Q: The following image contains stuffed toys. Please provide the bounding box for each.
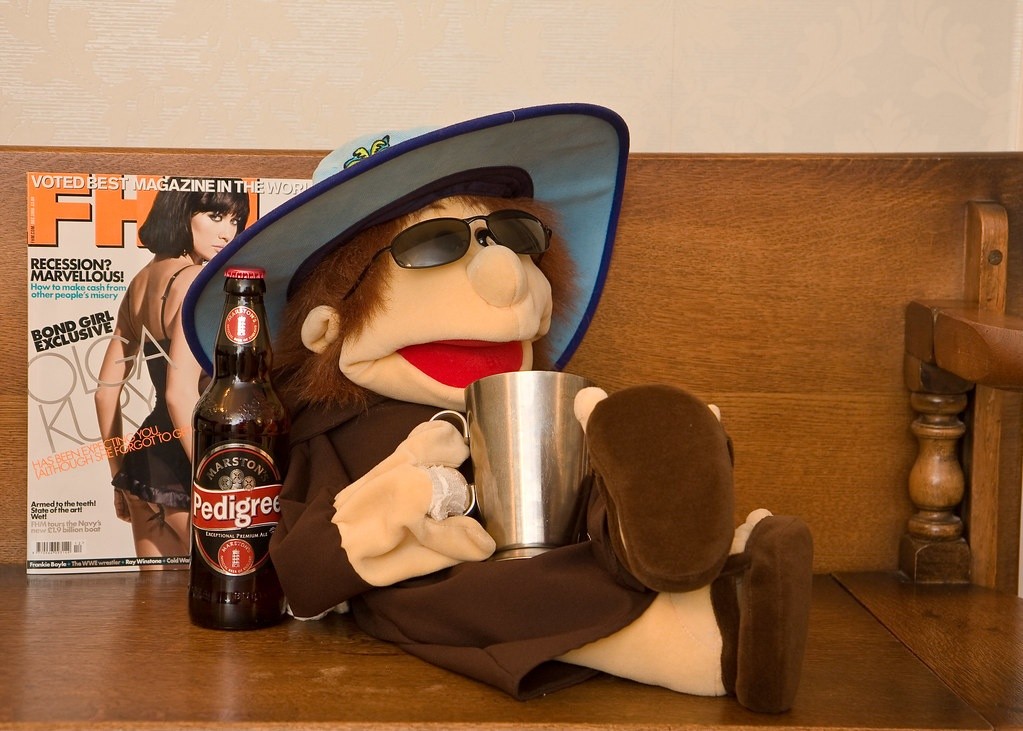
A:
[181,102,814,712]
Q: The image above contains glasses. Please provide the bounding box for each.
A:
[343,208,553,304]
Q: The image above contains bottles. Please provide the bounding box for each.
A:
[189,267,287,627]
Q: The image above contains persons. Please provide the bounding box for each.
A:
[95,177,251,571]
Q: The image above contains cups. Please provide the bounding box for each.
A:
[429,371,586,562]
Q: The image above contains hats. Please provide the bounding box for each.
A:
[180,104,630,379]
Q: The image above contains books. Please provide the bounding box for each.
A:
[27,171,311,574]
